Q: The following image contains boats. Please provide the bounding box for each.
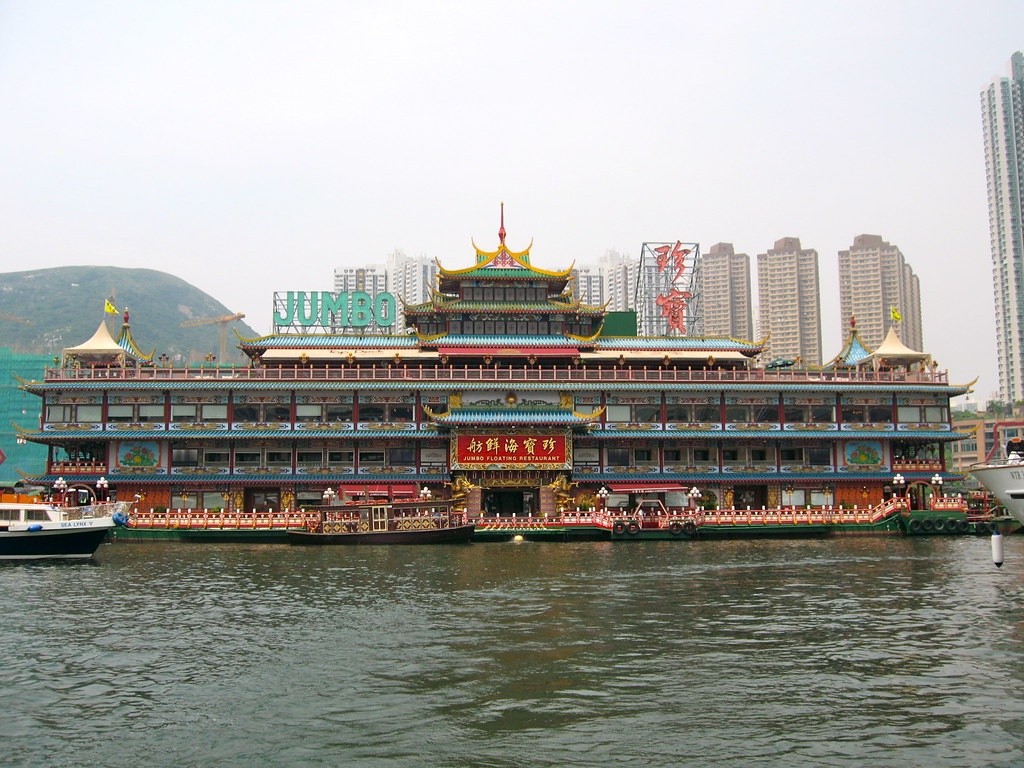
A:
[0,481,137,561]
[966,422,1024,526]
[286,463,475,545]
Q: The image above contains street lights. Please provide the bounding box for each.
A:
[688,487,703,513]
[322,486,335,505]
[53,477,68,506]
[96,477,109,500]
[931,474,943,499]
[893,473,905,499]
[596,487,610,512]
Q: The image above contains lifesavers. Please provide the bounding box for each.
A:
[613,521,626,534]
[113,512,126,525]
[958,520,969,531]
[627,522,639,535]
[988,522,999,533]
[683,523,696,536]
[976,522,987,534]
[946,518,958,531]
[922,519,933,531]
[909,519,921,530]
[934,519,945,530]
[670,523,682,534]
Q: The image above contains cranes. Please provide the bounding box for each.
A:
[178,314,243,363]
